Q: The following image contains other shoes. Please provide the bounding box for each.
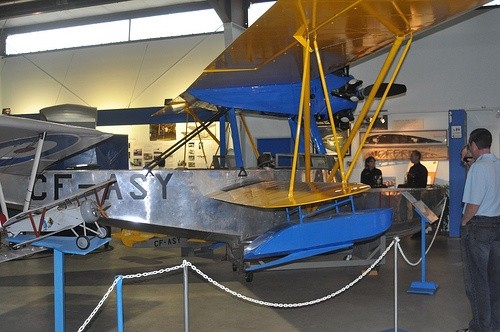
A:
[411,234,420,240]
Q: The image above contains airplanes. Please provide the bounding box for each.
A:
[0,0,492,284]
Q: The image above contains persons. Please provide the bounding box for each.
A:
[457,128,500,332]
[360,156,387,188]
[398,150,427,239]
